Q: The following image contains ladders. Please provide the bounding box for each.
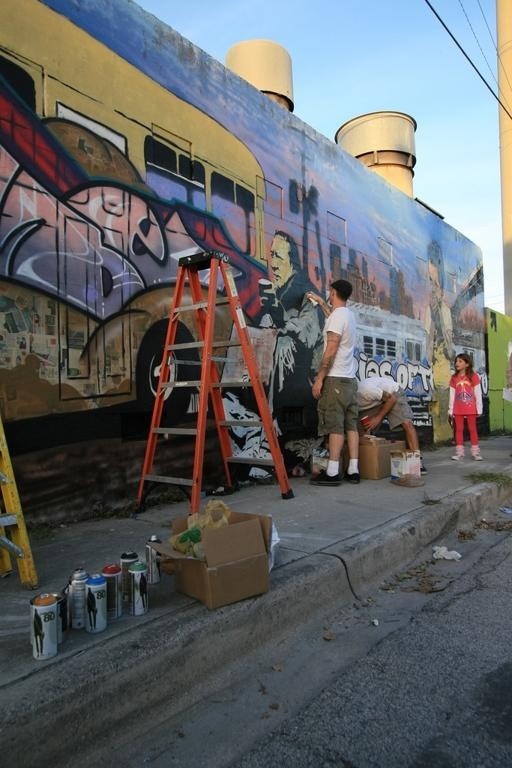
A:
[0,420,36,589]
[133,250,296,514]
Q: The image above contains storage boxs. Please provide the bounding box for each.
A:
[146,511,273,609]
[325,439,422,480]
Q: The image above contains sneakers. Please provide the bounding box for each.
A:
[344,469,360,484]
[310,469,342,486]
[421,465,427,474]
[474,454,483,460]
[452,453,464,460]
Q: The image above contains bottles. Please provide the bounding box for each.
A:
[28,529,163,660]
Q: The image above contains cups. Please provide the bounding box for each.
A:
[361,415,372,431]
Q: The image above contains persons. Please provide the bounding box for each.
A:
[356,377,429,475]
[257,229,326,442]
[446,352,485,461]
[423,239,458,367]
[305,279,361,486]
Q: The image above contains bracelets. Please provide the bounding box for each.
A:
[319,302,326,307]
